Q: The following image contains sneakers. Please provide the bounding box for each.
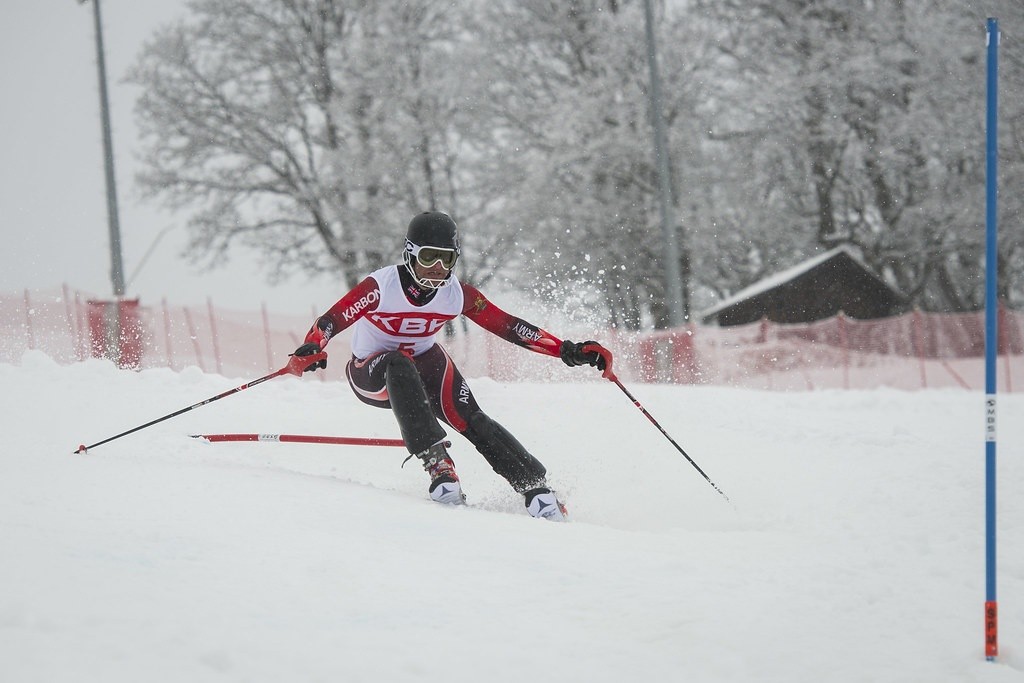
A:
[520,477,568,522]
[415,440,468,506]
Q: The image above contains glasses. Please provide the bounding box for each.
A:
[402,235,461,270]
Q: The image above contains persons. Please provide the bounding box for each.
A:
[287,209,612,524]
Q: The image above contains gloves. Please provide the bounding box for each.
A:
[560,340,607,371]
[288,344,327,372]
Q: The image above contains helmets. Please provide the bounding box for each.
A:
[405,211,459,267]
